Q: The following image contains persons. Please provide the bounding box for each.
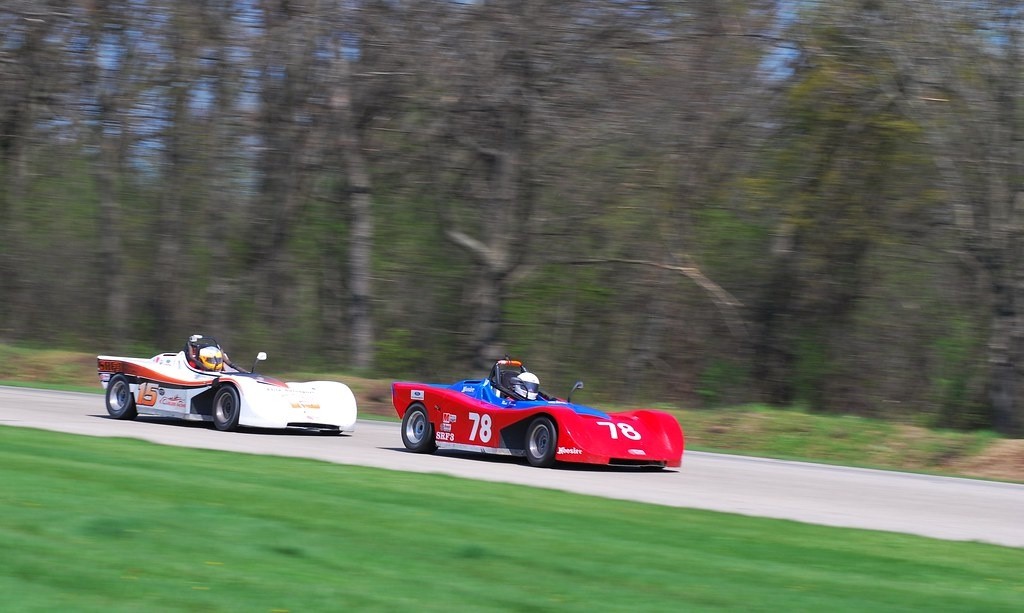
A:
[196,347,223,372]
[505,372,540,401]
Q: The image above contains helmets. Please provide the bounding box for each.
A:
[515,372,540,401]
[200,346,223,371]
